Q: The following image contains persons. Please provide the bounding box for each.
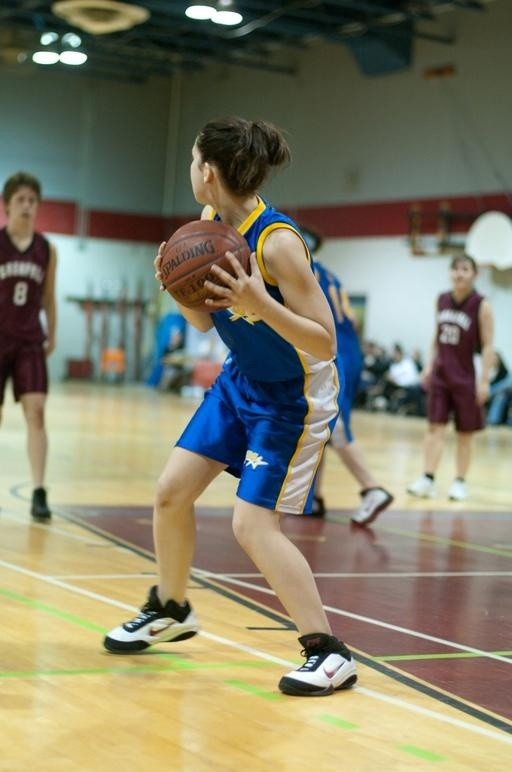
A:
[159,329,226,395]
[284,222,393,529]
[277,508,391,612]
[354,340,511,430]
[0,169,59,520]
[102,116,360,696]
[405,253,495,503]
[0,515,57,556]
[408,492,466,656]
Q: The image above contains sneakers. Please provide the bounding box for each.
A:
[104,583,201,654]
[407,476,437,499]
[294,494,327,518]
[32,488,53,521]
[447,482,467,502]
[276,631,358,697]
[351,485,394,529]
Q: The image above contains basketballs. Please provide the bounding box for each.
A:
[158,221,251,310]
